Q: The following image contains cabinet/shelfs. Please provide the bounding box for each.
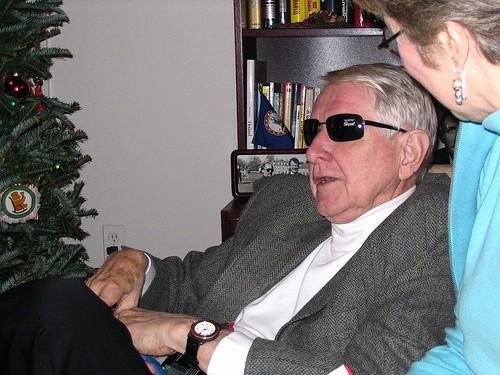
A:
[221,0,459,245]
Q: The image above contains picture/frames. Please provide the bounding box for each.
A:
[231,148,308,200]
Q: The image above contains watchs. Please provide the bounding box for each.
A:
[185,318,220,368]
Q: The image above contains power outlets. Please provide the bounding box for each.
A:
[102,224,126,262]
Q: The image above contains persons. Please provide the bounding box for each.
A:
[285,158,303,176]
[253,163,274,190]
[243,165,247,179]
[237,163,242,183]
[0,62,456,375]
[345,0,500,375]
[433,110,460,166]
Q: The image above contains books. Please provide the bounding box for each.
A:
[248,0,380,30]
[247,59,320,150]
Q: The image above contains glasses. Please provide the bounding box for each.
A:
[378,27,415,58]
[303,114,409,146]
